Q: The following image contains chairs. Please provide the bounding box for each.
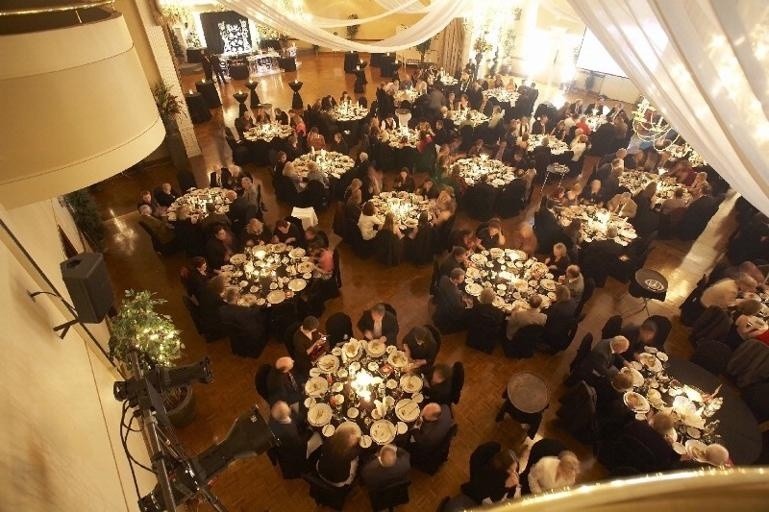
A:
[242,301,472,512]
[425,214,601,361]
[135,66,769,512]
[560,197,766,483]
[469,440,516,489]
[518,434,567,477]
[182,66,737,285]
[141,158,271,263]
[176,208,352,362]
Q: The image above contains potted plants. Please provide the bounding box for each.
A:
[107,287,195,429]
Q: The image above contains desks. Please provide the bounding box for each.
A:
[227,52,296,71]
[344,51,402,82]
[500,367,554,423]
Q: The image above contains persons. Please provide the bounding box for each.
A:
[582,320,731,470]
[138,51,629,339]
[438,448,580,510]
[628,114,713,232]
[701,261,768,350]
[267,315,453,500]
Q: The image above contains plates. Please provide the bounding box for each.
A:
[405,212,433,229]
[463,248,559,315]
[618,350,712,464]
[219,245,315,307]
[743,289,769,331]
[617,221,638,240]
[305,339,425,450]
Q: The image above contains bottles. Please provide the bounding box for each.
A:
[703,397,724,418]
[703,420,720,436]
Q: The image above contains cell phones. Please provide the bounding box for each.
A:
[319,336,327,341]
